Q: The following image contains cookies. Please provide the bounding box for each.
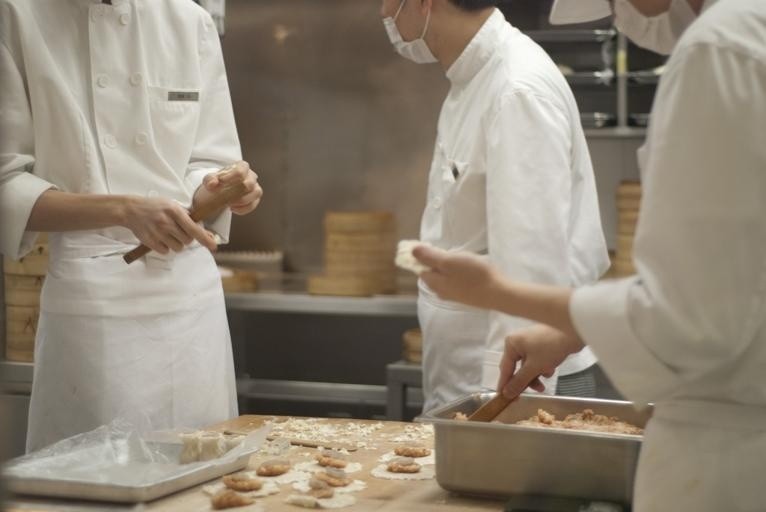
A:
[211,445,430,510]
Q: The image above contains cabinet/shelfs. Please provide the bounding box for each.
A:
[521,26,665,137]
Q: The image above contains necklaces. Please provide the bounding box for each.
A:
[410,2,766,510]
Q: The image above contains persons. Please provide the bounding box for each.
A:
[0,0,266,463]
[377,1,611,421]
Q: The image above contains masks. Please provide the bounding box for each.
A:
[382,0,440,64]
[612,0,677,56]
